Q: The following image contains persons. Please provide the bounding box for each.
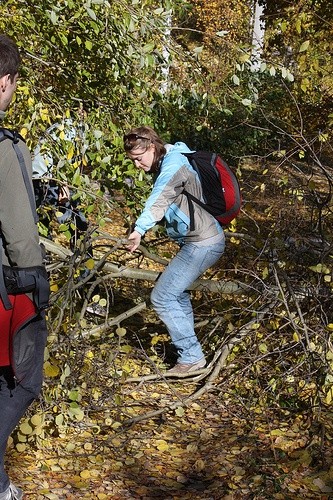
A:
[122,127,225,373]
[0,33,104,500]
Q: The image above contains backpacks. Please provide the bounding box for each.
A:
[158,149,242,226]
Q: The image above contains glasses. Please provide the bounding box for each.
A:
[123,134,153,143]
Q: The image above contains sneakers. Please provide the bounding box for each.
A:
[167,359,206,373]
[9,484,23,500]
[86,303,107,317]
